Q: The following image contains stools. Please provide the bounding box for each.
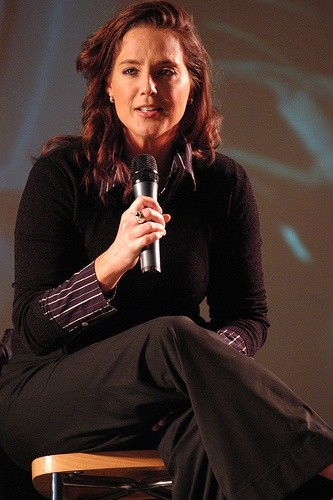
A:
[31,449,165,500]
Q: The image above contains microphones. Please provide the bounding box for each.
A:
[131,154,162,273]
